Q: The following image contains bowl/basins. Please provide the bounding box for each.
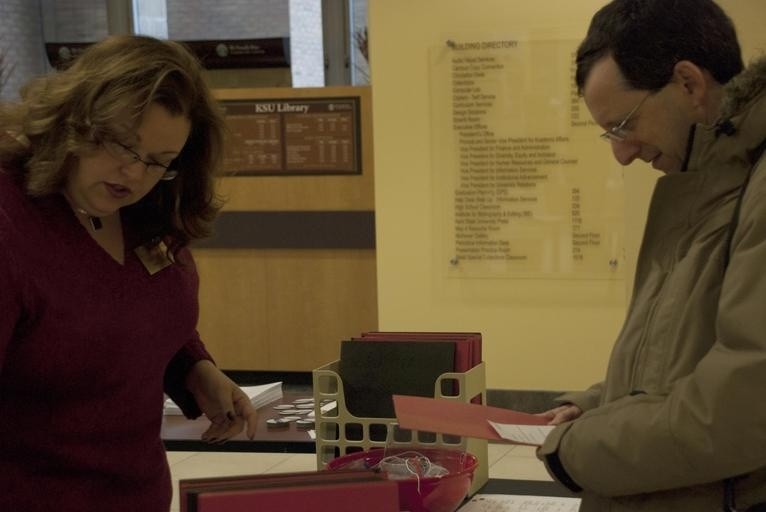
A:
[326,446,478,511]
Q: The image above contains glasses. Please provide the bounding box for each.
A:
[100,137,178,181]
[600,90,650,142]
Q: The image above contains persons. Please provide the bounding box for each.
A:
[530,0,766,512]
[0,34,260,511]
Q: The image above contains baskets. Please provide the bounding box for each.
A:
[312,359,489,499]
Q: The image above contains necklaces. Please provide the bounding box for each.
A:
[70,202,103,232]
